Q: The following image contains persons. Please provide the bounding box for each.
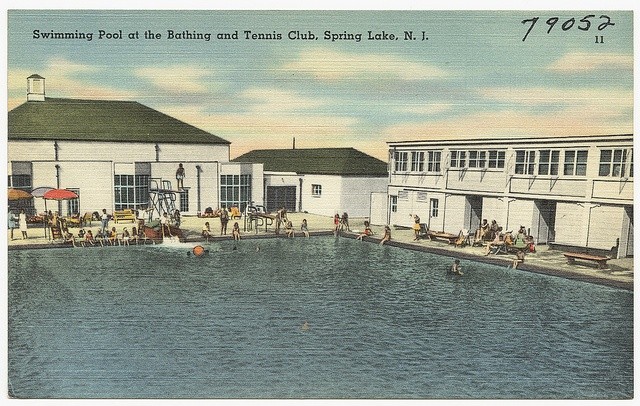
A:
[176,164,185,192]
[276,206,287,234]
[47,210,52,231]
[99,209,109,229]
[257,247,260,252]
[220,205,229,235]
[490,220,498,231]
[202,222,211,243]
[356,221,373,241]
[204,250,209,255]
[186,252,191,257]
[131,227,137,245]
[233,223,240,241]
[19,209,27,239]
[8,209,15,241]
[515,226,535,252]
[334,213,341,231]
[483,236,504,256]
[78,229,86,247]
[61,229,76,247]
[450,260,459,272]
[503,230,514,244]
[105,230,112,246]
[341,212,352,233]
[513,249,524,269]
[481,219,489,232]
[111,227,121,246]
[174,210,180,228]
[285,221,294,238]
[494,227,503,241]
[301,219,309,237]
[95,228,105,247]
[130,205,155,232]
[158,212,173,239]
[379,225,391,245]
[86,230,95,246]
[122,227,131,247]
[413,215,420,236]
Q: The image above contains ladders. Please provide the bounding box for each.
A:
[51,225,63,239]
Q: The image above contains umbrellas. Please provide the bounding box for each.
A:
[31,186,56,213]
[43,188,79,216]
[8,188,34,201]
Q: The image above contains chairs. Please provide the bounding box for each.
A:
[80,211,93,226]
[453,228,471,247]
[231,205,242,220]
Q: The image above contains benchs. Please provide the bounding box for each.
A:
[113,208,136,223]
[563,251,611,269]
[426,230,460,245]
